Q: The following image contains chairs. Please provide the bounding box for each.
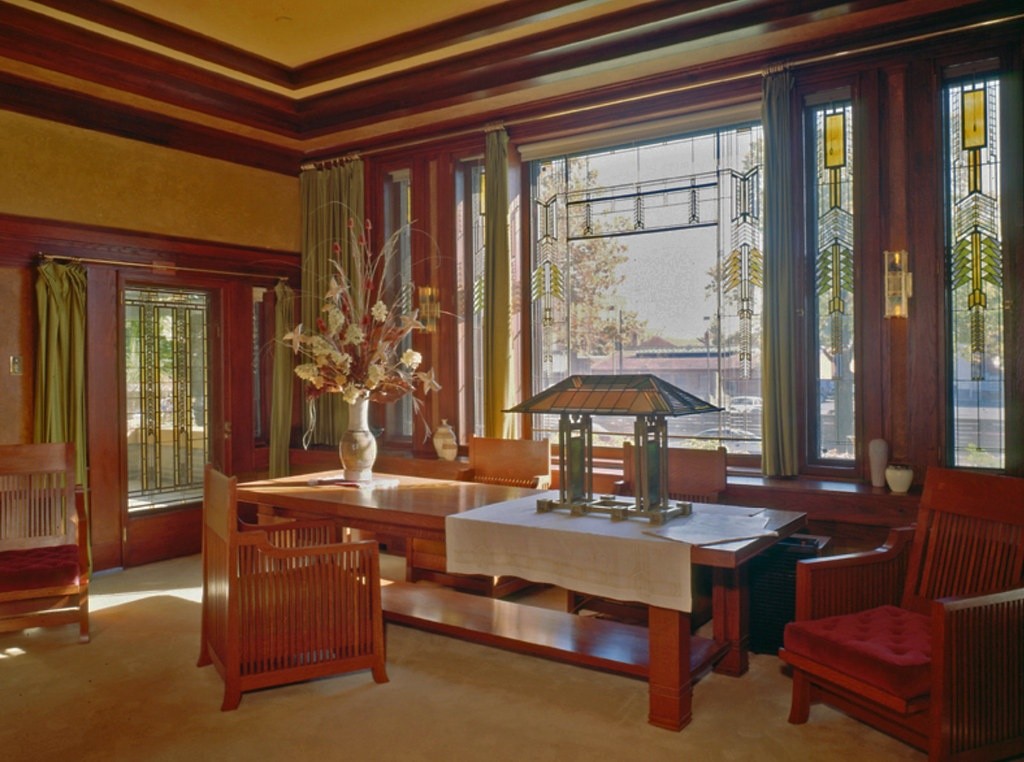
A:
[194,461,390,713]
[778,467,1024,762]
[565,442,728,637]
[0,442,92,646]
[405,432,553,599]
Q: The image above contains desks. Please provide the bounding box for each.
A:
[235,469,811,733]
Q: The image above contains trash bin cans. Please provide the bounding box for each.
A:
[749,536,820,655]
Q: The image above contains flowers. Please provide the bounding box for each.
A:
[279,201,463,451]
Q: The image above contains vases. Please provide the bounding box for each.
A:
[339,389,379,481]
[885,464,914,493]
[870,438,888,487]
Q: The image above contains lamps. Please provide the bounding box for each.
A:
[417,286,438,334]
[883,250,913,318]
